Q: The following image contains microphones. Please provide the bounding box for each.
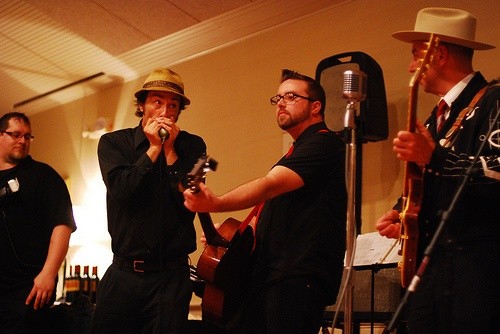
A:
[342,69,368,100]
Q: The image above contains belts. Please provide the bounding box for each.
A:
[113,253,151,273]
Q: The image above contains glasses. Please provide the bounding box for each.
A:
[4,131,34,142]
[270,91,314,105]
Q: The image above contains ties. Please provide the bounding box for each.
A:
[435,100,448,134]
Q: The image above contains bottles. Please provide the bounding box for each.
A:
[373,323,386,334]
[64,265,100,304]
[359,323,371,334]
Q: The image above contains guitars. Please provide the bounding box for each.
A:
[397,33,439,290]
[184,152,256,330]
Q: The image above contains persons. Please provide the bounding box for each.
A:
[92,68,206,334]
[0,112,77,334]
[183,70,348,334]
[376,9,500,334]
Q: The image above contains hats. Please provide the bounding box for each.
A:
[135,68,190,108]
[391,7,496,55]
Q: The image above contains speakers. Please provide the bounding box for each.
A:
[315,51,388,145]
